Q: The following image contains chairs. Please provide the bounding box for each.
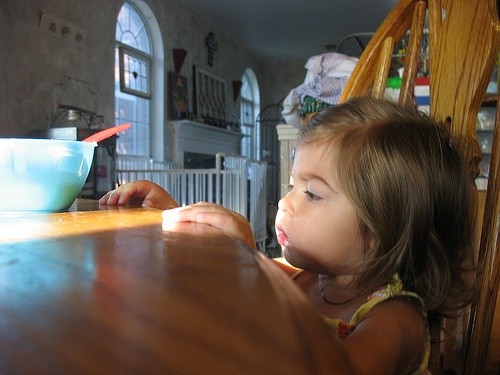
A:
[338,0,500,375]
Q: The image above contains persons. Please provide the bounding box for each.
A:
[98,97,479,375]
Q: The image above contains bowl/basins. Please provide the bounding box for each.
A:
[0,138,94,211]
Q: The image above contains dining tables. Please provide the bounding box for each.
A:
[0,197,352,375]
